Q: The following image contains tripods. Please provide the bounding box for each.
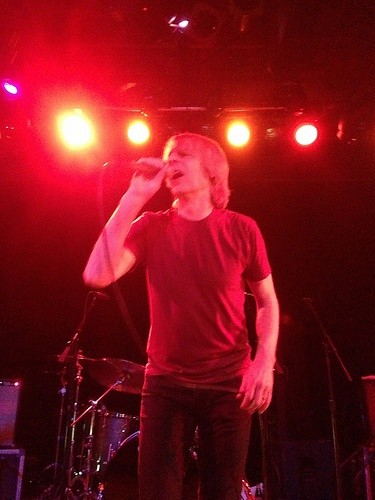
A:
[38,301,129,500]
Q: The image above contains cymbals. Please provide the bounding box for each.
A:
[85,359,146,394]
[56,354,94,364]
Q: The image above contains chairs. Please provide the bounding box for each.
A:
[0,382,25,500]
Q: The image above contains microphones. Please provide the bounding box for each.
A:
[303,297,327,337]
[106,161,174,177]
[89,291,111,301]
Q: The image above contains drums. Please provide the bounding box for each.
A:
[65,400,106,459]
[110,431,201,500]
[93,410,140,464]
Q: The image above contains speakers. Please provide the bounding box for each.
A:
[0,449,25,500]
[0,381,21,449]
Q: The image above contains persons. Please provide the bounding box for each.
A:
[83,133,279,500]
